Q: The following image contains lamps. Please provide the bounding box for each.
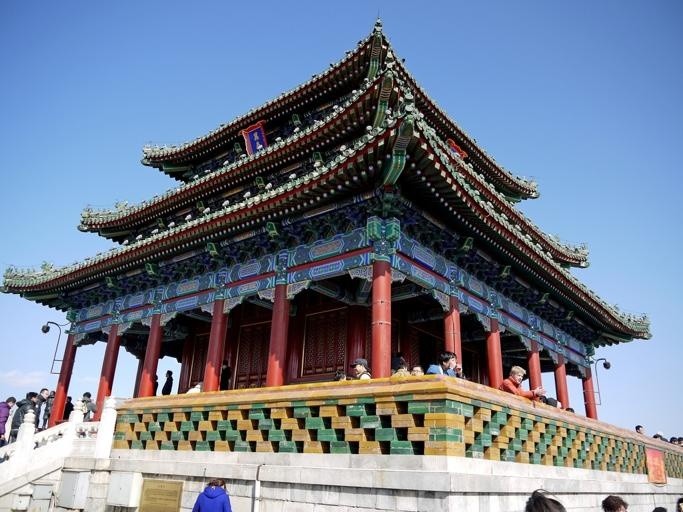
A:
[590,356,610,406]
[41,321,72,374]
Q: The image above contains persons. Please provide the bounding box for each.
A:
[191,476,234,512]
[219,360,232,390]
[523,487,567,512]
[652,434,661,440]
[154,375,160,397]
[675,497,683,512]
[337,357,372,381]
[0,385,102,463]
[677,437,682,447]
[599,494,630,512]
[161,370,174,396]
[390,351,578,413]
[634,425,644,435]
[669,437,677,446]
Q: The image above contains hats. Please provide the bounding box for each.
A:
[350,358,368,368]
